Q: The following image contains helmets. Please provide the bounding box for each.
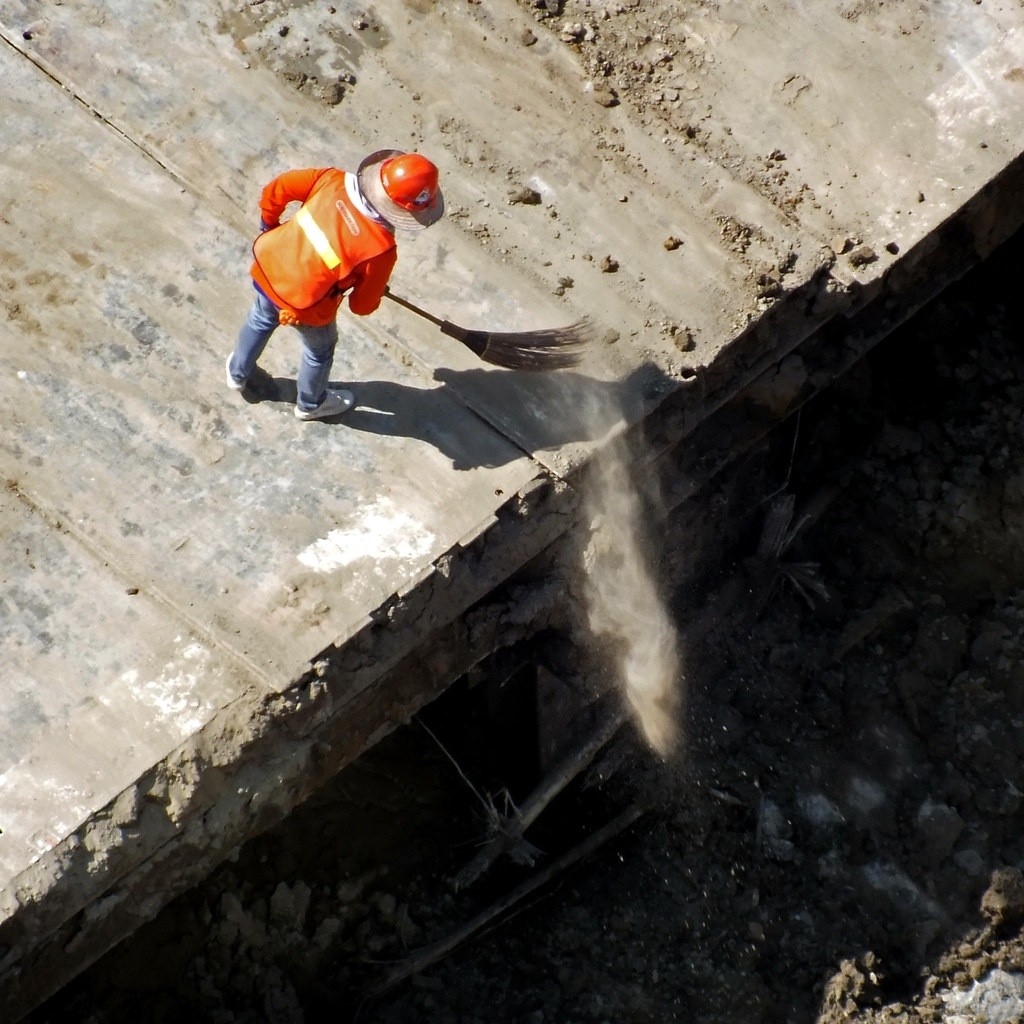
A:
[381,153,438,211]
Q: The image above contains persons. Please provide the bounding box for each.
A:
[223,147,444,421]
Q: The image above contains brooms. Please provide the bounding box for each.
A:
[382,289,594,374]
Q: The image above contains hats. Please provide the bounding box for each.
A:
[356,147,444,231]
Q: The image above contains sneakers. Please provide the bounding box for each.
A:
[293,389,355,421]
[226,351,246,392]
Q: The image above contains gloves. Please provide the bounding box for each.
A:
[261,218,281,232]
